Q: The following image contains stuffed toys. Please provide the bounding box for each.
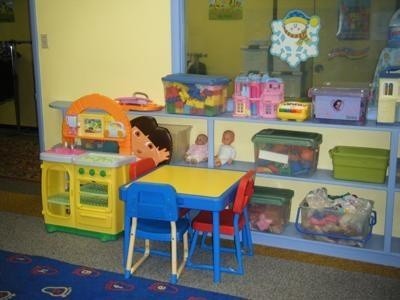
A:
[253,143,312,178]
[249,203,291,234]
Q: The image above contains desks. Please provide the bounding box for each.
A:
[119,164,248,283]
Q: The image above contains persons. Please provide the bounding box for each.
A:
[214,130,236,167]
[184,133,209,164]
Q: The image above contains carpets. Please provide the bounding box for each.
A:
[0,248,249,300]
[0,131,41,183]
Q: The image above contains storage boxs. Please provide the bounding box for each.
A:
[329,144,390,184]
[155,123,192,163]
[162,41,369,124]
[251,129,323,179]
[294,194,376,248]
[246,185,294,234]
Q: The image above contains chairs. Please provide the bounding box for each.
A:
[122,181,190,285]
[186,171,255,275]
[1,44,22,131]
[130,158,198,224]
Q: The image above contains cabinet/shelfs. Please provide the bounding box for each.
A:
[49,101,400,279]
